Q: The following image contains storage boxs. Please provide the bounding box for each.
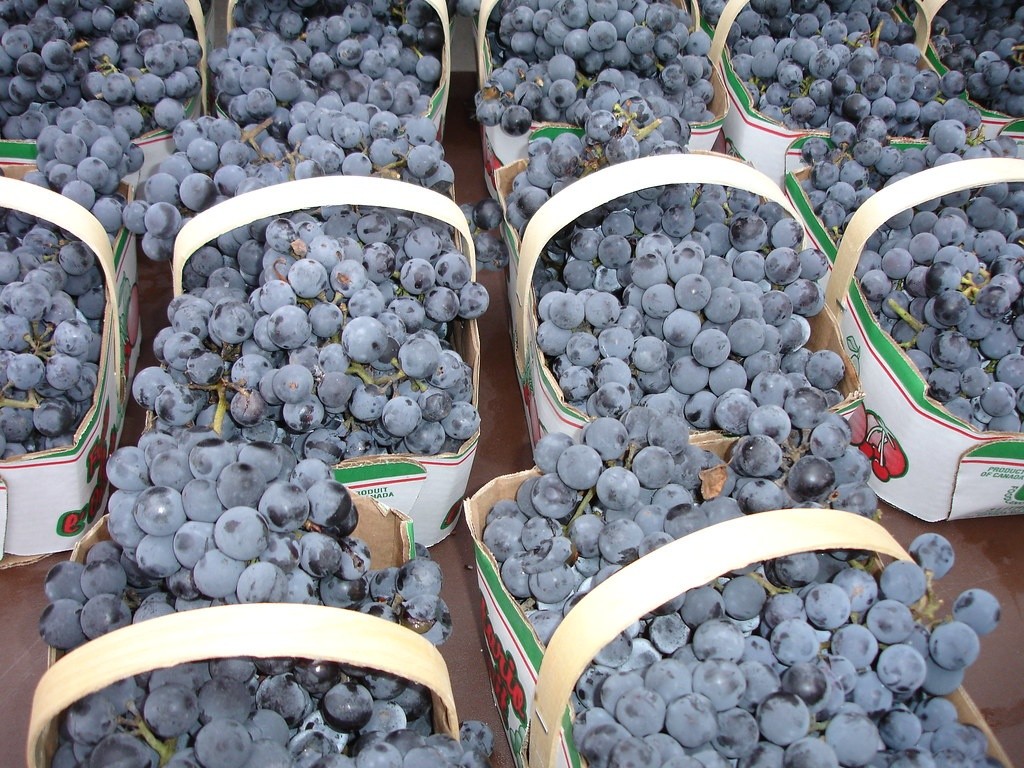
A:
[1,0,1023,767]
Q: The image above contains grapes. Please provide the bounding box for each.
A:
[0,0,1024,768]
